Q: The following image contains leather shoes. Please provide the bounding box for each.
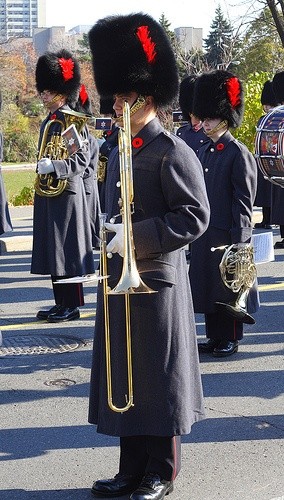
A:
[91,476,136,500]
[130,474,173,500]
[197,339,215,353]
[47,305,80,322]
[275,239,284,248]
[212,341,239,356]
[254,221,272,229]
[36,304,62,320]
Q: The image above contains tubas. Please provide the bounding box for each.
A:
[34,109,95,197]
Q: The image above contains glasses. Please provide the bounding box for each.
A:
[39,91,55,97]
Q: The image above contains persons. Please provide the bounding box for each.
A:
[0,92,13,237]
[274,71,284,249]
[178,75,209,154]
[193,69,258,357]
[253,80,277,230]
[76,83,102,248]
[29,48,91,324]
[87,11,210,500]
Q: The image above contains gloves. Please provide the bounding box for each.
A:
[37,158,54,174]
[233,243,250,251]
[104,222,125,257]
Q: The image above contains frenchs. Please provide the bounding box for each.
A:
[211,243,257,324]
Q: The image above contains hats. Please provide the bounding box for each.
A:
[194,70,244,128]
[178,75,200,113]
[88,12,179,106]
[68,83,93,116]
[99,96,116,114]
[35,50,81,103]
[272,72,284,104]
[260,79,276,106]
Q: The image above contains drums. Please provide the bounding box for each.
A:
[255,105,284,188]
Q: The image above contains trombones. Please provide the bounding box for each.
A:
[100,101,159,414]
